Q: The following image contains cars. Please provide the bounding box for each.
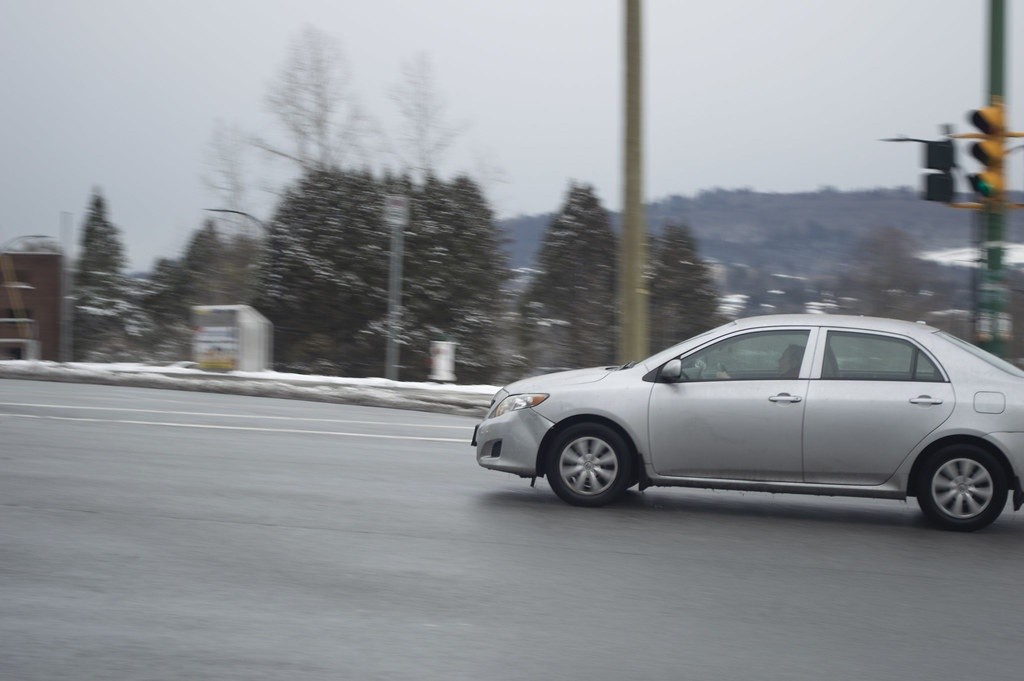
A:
[469,314,1024,532]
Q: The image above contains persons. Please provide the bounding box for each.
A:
[715,344,804,379]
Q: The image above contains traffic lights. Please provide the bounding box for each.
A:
[922,136,952,202]
[969,105,1004,198]
